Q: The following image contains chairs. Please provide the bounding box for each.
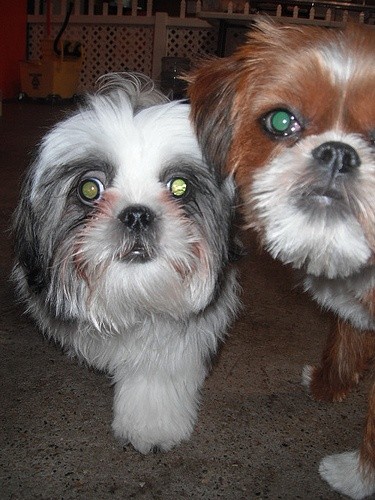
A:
[159,56,193,100]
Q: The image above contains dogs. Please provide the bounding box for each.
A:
[5,10,375,500]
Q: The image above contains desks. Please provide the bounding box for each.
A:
[196,11,345,58]
